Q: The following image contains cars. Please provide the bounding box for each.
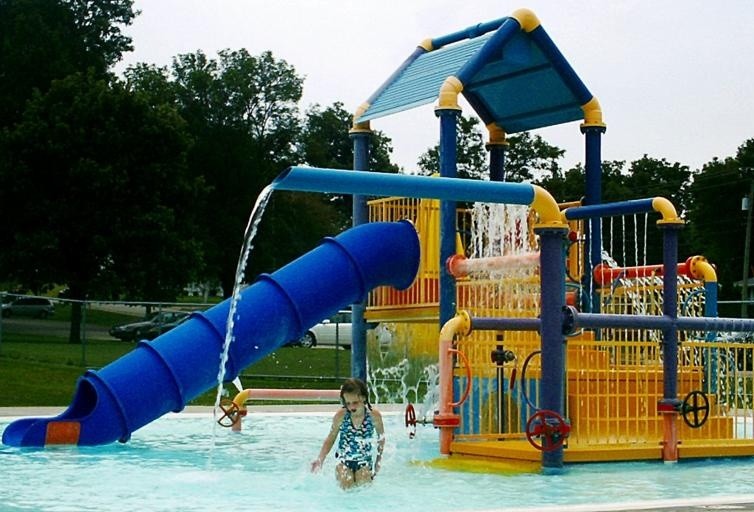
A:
[660,332,754,374]
[0,296,55,318]
[108,311,191,341]
[298,310,352,349]
[59,288,88,300]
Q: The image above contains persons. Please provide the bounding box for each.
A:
[310,377,386,491]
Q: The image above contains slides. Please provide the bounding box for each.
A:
[2,222,420,447]
